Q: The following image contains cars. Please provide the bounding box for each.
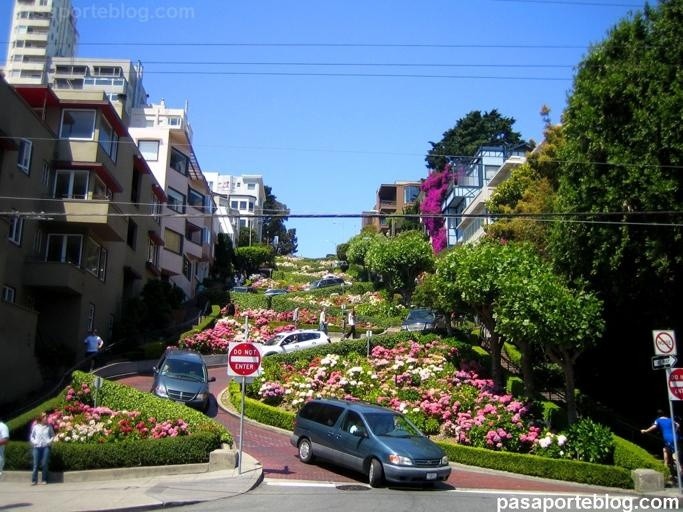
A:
[309,277,344,290]
[263,288,288,297]
[259,327,332,357]
[401,306,446,336]
[333,260,348,267]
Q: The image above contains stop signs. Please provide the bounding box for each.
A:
[226,341,262,377]
[665,366,683,401]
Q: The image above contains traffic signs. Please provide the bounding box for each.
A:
[650,354,678,372]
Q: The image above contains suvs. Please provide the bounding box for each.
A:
[149,345,217,411]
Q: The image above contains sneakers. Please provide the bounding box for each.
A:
[31,481,47,485]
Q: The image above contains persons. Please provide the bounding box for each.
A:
[318,306,330,335]
[30,411,56,485]
[351,419,367,436]
[640,410,683,475]
[84,328,104,372]
[0,417,10,473]
[293,304,301,329]
[343,308,356,339]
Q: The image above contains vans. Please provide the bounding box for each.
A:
[288,399,451,488]
